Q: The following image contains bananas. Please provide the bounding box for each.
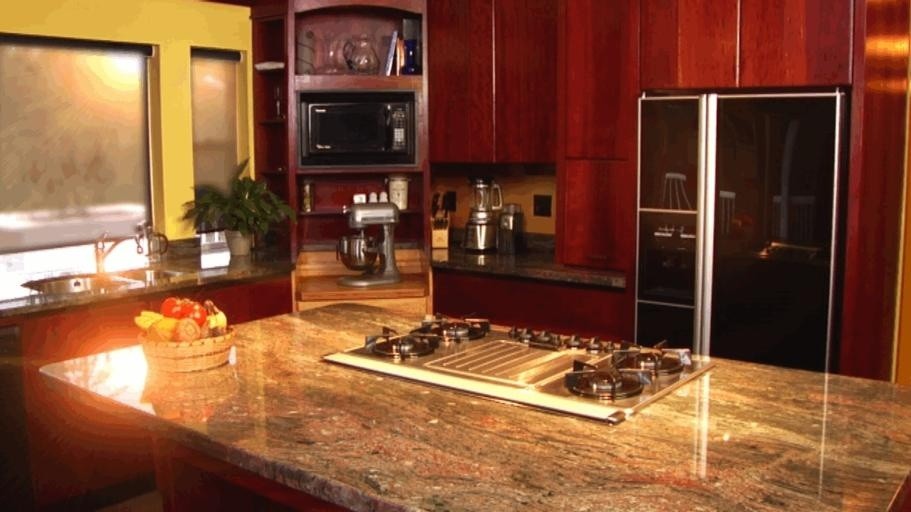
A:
[133,299,227,338]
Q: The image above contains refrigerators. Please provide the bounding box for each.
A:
[632,83,849,377]
[606,350,842,512]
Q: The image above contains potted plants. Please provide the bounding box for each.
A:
[180,156,298,256]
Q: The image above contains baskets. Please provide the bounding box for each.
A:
[139,327,236,373]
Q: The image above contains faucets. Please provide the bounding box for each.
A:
[95,231,146,271]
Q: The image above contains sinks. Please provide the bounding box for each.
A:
[22,274,127,296]
[114,266,188,284]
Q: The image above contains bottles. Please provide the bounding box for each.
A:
[499,203,521,254]
[296,30,315,74]
[403,38,419,75]
[299,178,315,212]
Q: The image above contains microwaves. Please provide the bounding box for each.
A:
[305,99,413,164]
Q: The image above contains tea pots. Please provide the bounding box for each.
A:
[342,33,379,75]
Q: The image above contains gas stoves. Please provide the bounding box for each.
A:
[321,311,718,424]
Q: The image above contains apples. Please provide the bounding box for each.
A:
[160,296,206,325]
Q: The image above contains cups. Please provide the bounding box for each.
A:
[387,175,412,210]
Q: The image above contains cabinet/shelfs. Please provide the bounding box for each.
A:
[555,1,642,273]
[639,0,851,88]
[17,273,292,510]
[253,0,433,315]
[425,1,554,166]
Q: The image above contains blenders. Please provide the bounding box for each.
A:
[460,175,504,252]
[337,201,402,287]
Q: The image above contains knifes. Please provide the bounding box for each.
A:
[431,193,452,231]
